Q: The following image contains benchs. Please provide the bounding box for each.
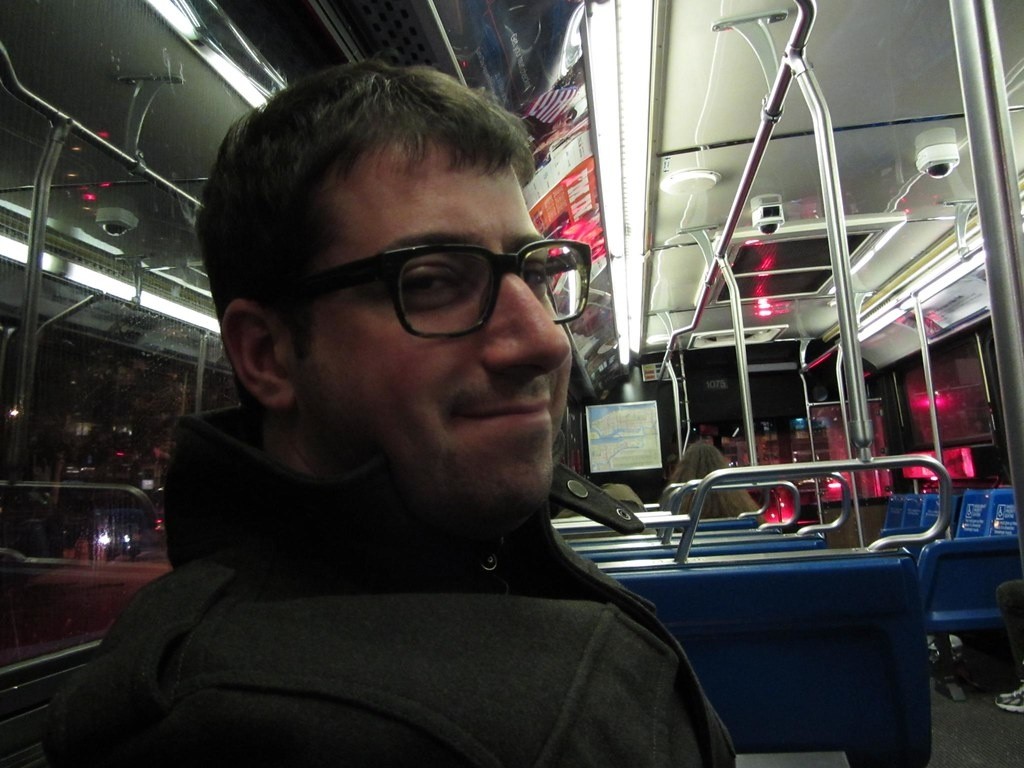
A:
[548,453,1024,767]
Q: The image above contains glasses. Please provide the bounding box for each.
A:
[270,234,593,340]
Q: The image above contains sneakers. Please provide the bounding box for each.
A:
[994,682,1024,713]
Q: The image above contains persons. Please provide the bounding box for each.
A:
[43,61,735,768]
[29,450,70,511]
[995,580,1024,714]
[663,440,764,526]
